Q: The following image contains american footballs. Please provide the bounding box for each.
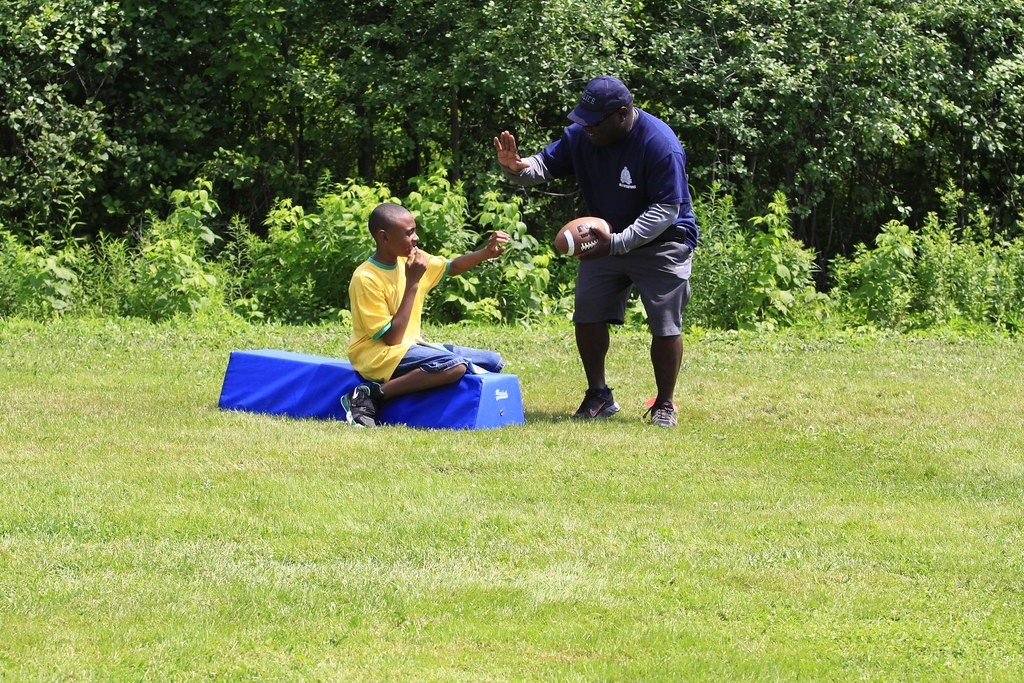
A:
[555,217,614,258]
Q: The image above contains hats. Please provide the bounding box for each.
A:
[568,75,633,126]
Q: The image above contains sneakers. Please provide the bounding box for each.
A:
[342,381,385,429]
[572,386,620,420]
[651,398,678,429]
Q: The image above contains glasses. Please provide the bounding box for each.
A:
[589,108,619,126]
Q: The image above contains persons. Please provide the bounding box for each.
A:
[339,201,509,431]
[494,75,699,429]
